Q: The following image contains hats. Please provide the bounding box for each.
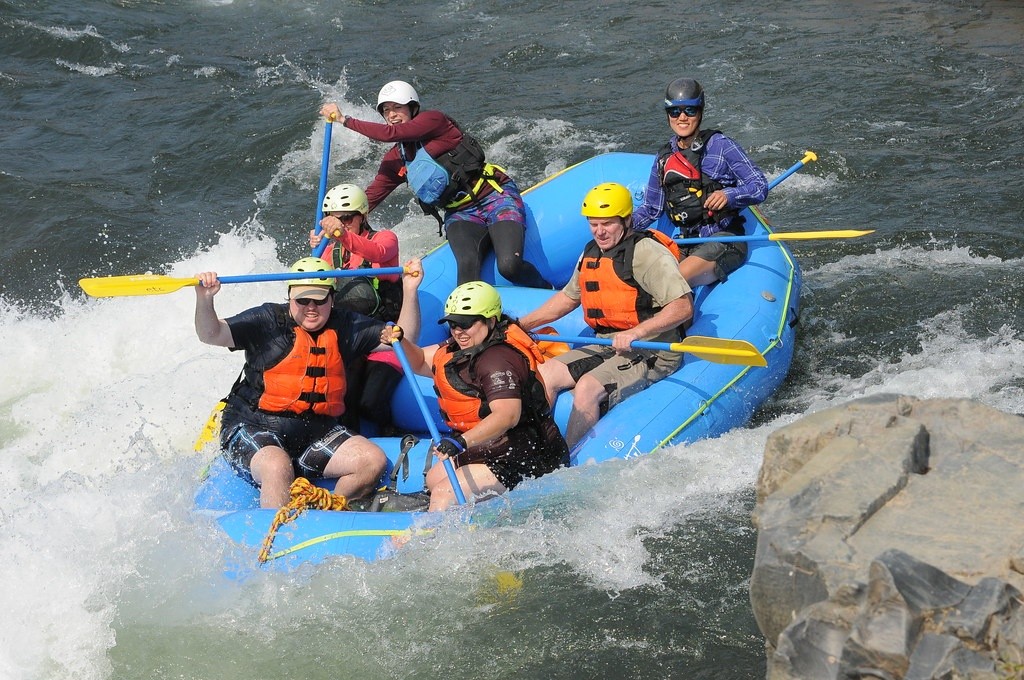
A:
[438,313,486,328]
[289,284,332,300]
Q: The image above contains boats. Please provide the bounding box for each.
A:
[189,149,805,584]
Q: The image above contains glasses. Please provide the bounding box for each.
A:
[340,212,359,225]
[449,321,481,330]
[297,297,330,306]
[665,107,701,118]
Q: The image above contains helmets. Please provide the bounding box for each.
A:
[288,257,335,291]
[444,280,501,322]
[664,77,704,109]
[582,181,633,219]
[376,80,420,117]
[322,184,369,217]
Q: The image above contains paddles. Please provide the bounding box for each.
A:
[79,266,419,298]
[528,333,767,367]
[390,324,468,508]
[768,152,818,189]
[311,113,336,259]
[313,223,340,258]
[672,229,875,244]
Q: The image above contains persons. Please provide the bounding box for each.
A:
[629,77,769,332]
[309,81,556,292]
[517,183,694,451]
[194,257,424,508]
[314,184,409,439]
[380,280,572,513]
